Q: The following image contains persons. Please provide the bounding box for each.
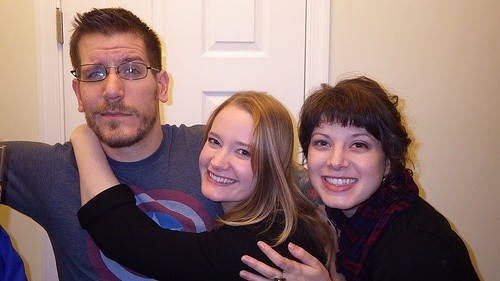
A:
[69,91,338,281]
[0,7,328,281]
[239,74,481,281]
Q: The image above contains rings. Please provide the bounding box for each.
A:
[274,276,286,281]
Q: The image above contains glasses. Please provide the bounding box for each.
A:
[70,62,162,83]
